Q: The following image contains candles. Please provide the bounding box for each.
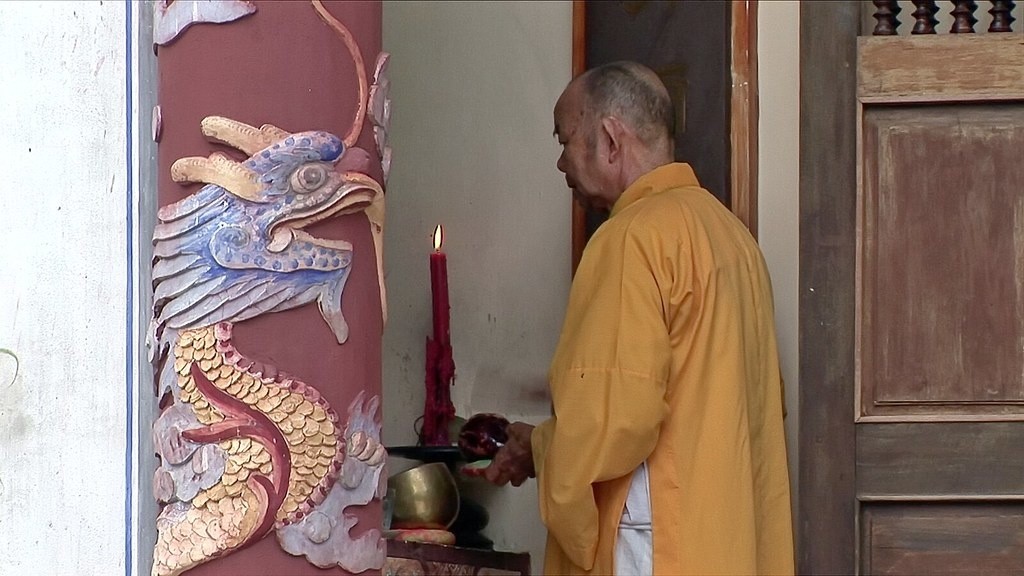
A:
[421,223,454,448]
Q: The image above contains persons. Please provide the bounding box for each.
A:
[482,60,798,576]
